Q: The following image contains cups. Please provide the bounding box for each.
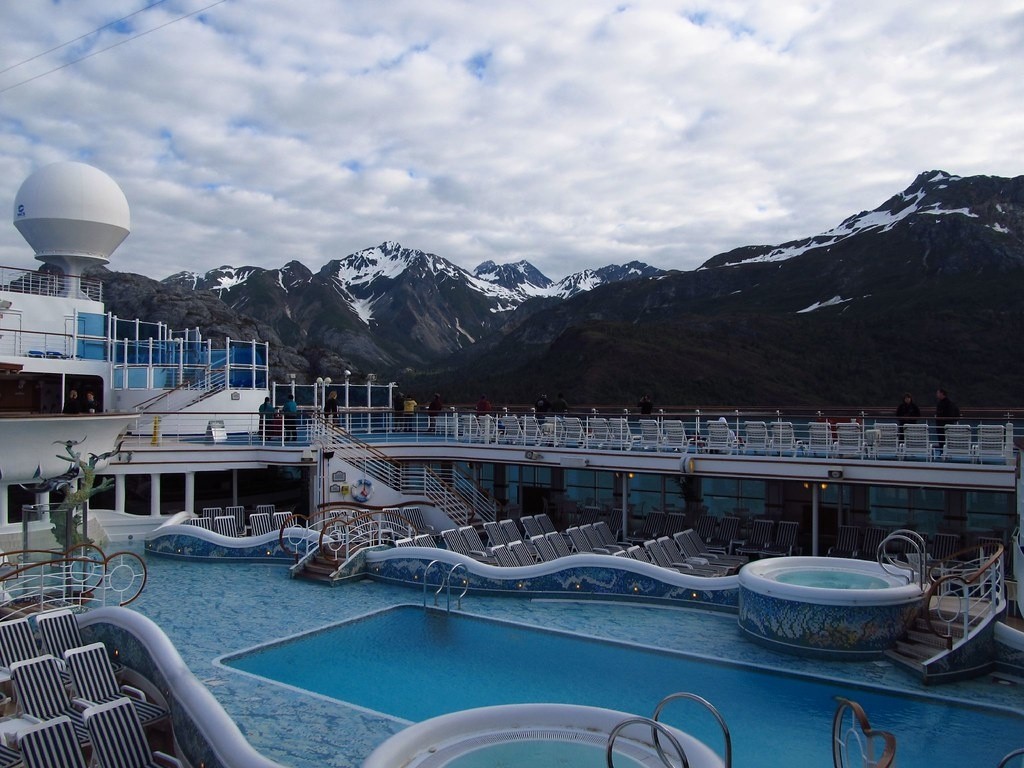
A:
[90,408,95,413]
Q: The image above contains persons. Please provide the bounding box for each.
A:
[324,391,341,444]
[936,390,955,458]
[534,394,551,424]
[552,393,569,416]
[259,397,275,440]
[283,395,297,441]
[896,394,921,444]
[427,395,441,432]
[84,392,101,413]
[637,395,653,414]
[69,390,83,414]
[477,395,491,426]
[395,394,417,431]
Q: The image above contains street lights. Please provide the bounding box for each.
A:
[317,377,331,434]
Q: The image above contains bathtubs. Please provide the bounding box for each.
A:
[737,556,932,660]
[358,702,728,767]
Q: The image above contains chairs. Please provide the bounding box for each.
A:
[332,506,1010,579]
[190,501,297,539]
[459,414,1004,461]
[0,609,180,768]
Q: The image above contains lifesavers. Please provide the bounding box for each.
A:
[351,479,374,502]
